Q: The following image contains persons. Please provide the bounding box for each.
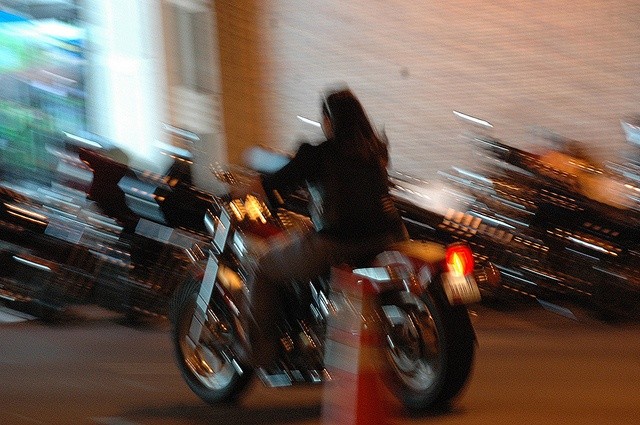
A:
[229,89,410,366]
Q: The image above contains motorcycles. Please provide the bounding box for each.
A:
[169,164,501,413]
